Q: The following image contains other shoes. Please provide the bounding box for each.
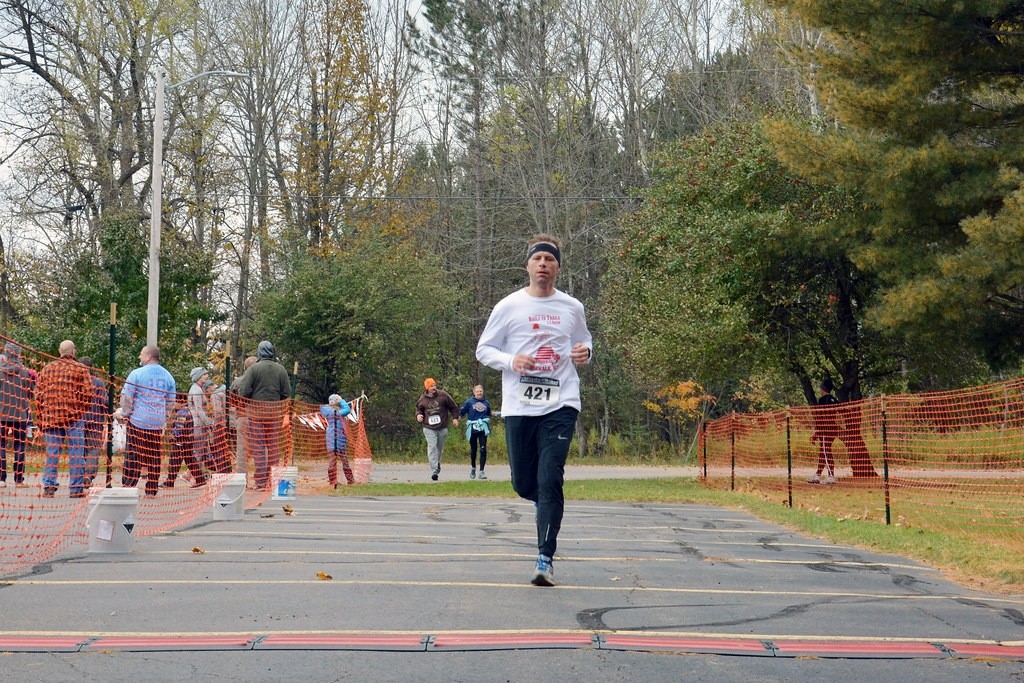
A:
[180,472,194,487]
[437,463,441,473]
[189,481,208,489]
[478,471,488,479]
[158,483,173,490]
[0,469,160,500]
[432,475,438,480]
[203,471,211,481]
[470,467,476,479]
[248,484,271,492]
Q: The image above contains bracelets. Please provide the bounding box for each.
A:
[588,347,590,358]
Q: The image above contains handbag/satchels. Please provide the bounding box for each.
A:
[103,418,127,454]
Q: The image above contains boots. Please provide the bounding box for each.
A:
[328,469,337,489]
[343,469,362,485]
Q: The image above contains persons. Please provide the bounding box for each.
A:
[114,345,176,498]
[415,378,459,480]
[240,340,292,491]
[35,340,91,497]
[78,356,108,488]
[204,380,231,472]
[320,393,355,489]
[157,393,206,489]
[232,356,268,489]
[180,367,214,487]
[475,234,594,587]
[457,385,501,479]
[0,344,37,487]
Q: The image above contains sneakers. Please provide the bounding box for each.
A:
[531,554,556,586]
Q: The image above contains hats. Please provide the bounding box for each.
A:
[190,367,209,383]
[244,357,258,370]
[4,343,20,358]
[78,356,93,367]
[201,380,213,391]
[424,378,436,390]
[329,394,342,405]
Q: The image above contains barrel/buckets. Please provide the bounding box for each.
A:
[211,473,246,521]
[271,466,298,500]
[85,487,139,553]
[353,457,372,483]
